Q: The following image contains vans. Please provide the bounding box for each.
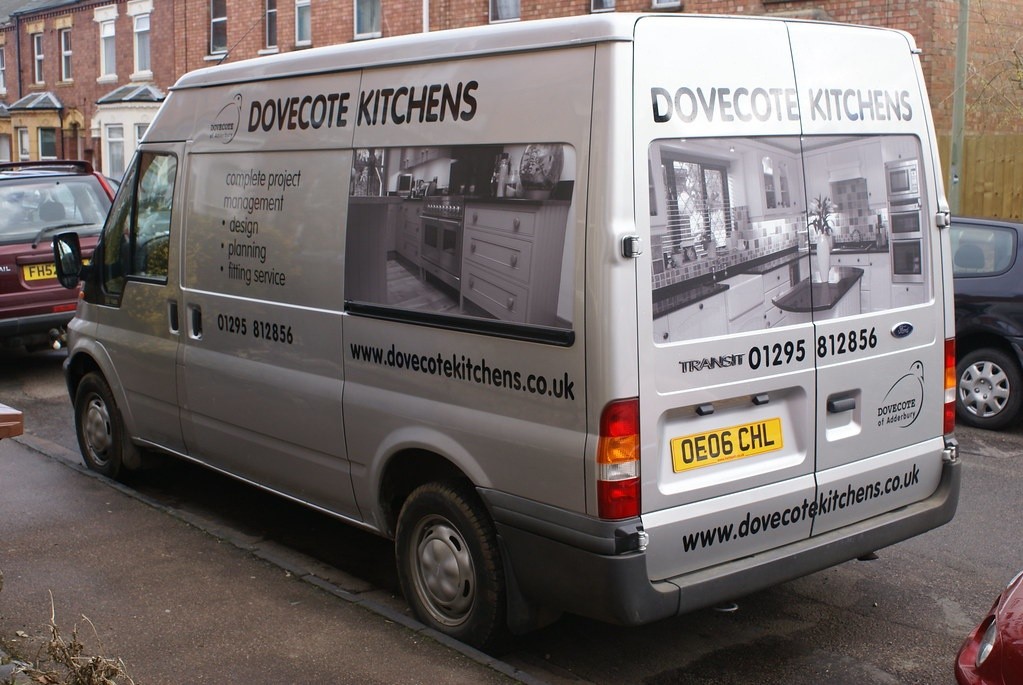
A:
[50,8,962,652]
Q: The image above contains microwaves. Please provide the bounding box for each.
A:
[883,159,921,205]
[396,173,416,196]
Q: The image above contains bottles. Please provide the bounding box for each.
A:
[495,152,510,198]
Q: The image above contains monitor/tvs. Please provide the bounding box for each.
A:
[397,174,413,195]
[449,147,504,196]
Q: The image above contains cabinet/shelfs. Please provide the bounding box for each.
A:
[345,194,572,326]
[653,156,924,340]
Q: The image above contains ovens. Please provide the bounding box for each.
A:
[418,195,472,303]
[887,199,923,240]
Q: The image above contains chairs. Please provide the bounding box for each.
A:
[39,202,66,221]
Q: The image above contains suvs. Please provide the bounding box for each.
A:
[940,219,1021,429]
[1,155,120,364]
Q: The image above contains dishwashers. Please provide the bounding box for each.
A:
[888,238,925,286]
